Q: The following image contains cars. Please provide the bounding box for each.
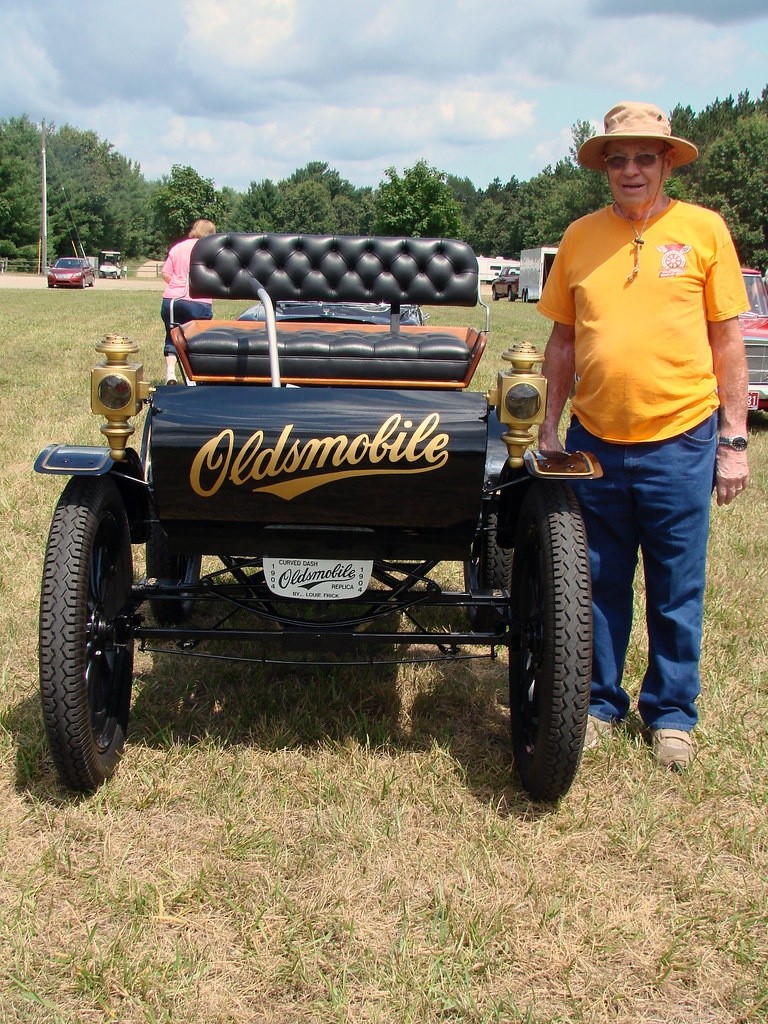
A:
[98,250,123,280]
[492,265,520,301]
[47,257,97,289]
[732,268,768,412]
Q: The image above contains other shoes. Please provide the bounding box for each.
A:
[583,715,611,750]
[650,728,692,768]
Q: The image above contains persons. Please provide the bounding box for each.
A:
[542,100,751,777]
[160,219,220,386]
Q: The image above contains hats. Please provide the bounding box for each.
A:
[579,100,698,170]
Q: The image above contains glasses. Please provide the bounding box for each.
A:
[604,151,664,169]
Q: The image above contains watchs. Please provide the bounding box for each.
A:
[719,434,753,453]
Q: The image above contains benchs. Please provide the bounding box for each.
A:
[160,236,489,387]
